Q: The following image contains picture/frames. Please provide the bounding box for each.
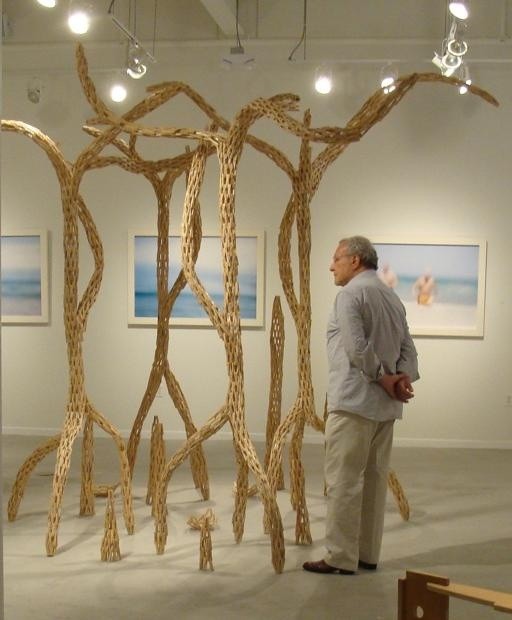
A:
[127,231,265,327]
[369,237,487,337]
[0,228,49,324]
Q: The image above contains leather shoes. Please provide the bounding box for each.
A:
[358,561,378,570]
[303,560,356,575]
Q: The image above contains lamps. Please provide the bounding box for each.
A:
[430,0,469,78]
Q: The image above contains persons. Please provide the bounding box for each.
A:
[414,270,437,306]
[302,235,420,573]
[378,262,399,290]
